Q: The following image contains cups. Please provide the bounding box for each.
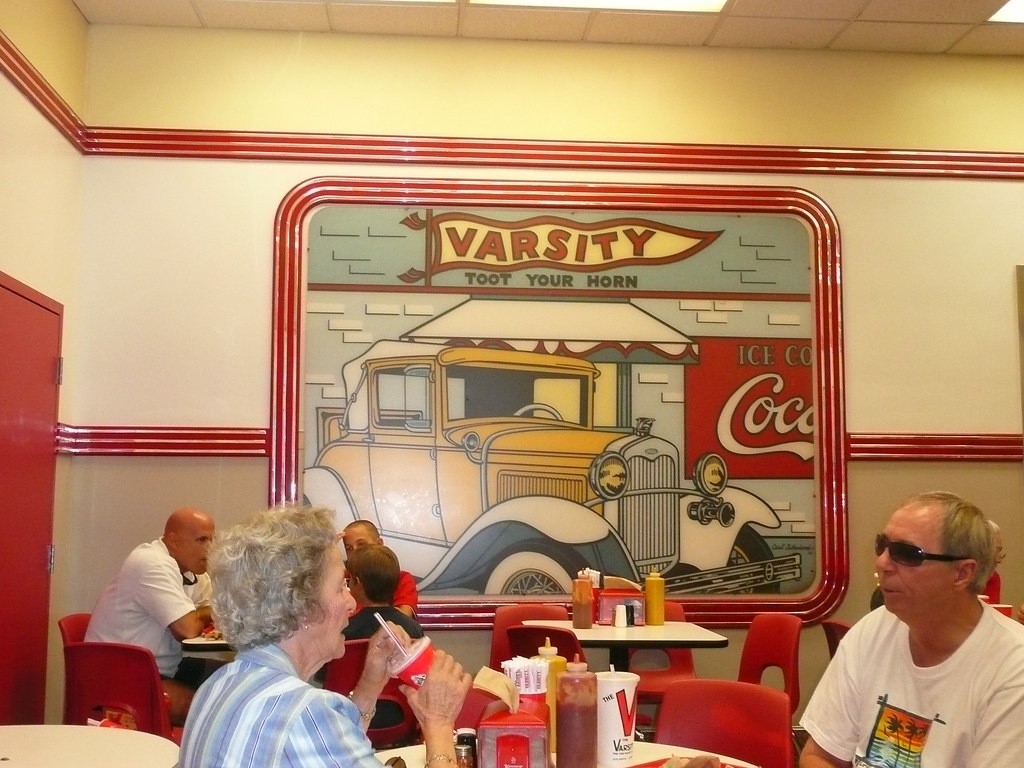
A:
[389,635,436,691]
[614,605,626,627]
[592,588,599,623]
[594,672,640,768]
[457,733,477,768]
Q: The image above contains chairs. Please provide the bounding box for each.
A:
[486,593,855,768]
[51,611,418,753]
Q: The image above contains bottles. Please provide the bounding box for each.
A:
[645,572,665,625]
[530,637,567,753]
[626,599,644,627]
[454,745,473,768]
[572,573,592,629]
[556,653,597,768]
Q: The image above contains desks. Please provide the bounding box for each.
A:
[519,616,728,743]
[0,724,183,768]
[180,633,237,652]
[372,740,761,768]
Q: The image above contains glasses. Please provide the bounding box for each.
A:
[875,534,968,566]
